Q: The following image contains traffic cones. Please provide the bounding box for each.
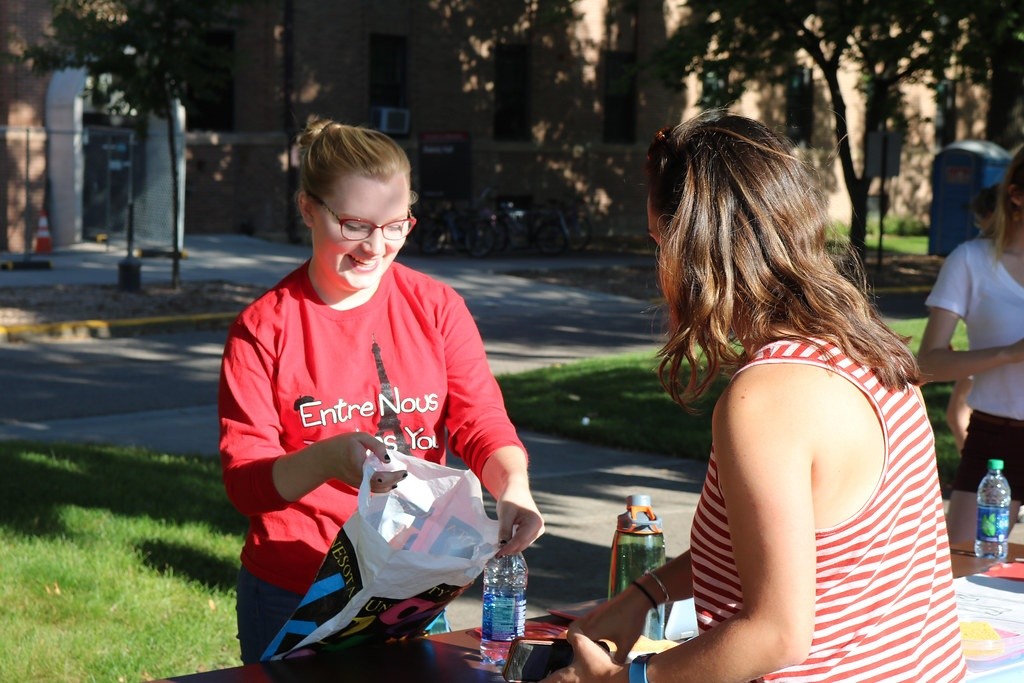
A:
[33,207,53,256]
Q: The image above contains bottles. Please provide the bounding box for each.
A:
[608,495,665,641]
[975,460,1010,560]
[480,552,528,668]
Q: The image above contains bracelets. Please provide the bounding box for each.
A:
[629,652,658,683]
[646,570,670,603]
[628,579,658,614]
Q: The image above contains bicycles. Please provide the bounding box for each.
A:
[409,193,593,261]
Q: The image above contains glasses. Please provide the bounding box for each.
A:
[307,193,417,242]
[646,127,684,172]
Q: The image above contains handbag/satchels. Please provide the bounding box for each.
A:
[255,444,520,661]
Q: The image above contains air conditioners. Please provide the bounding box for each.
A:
[373,106,411,134]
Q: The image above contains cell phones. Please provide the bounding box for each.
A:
[503,636,616,683]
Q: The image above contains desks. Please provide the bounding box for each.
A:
[149,559,1024,683]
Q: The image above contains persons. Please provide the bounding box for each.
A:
[216,115,546,663]
[538,108,968,683]
[918,162,1024,580]
[947,185,1005,457]
